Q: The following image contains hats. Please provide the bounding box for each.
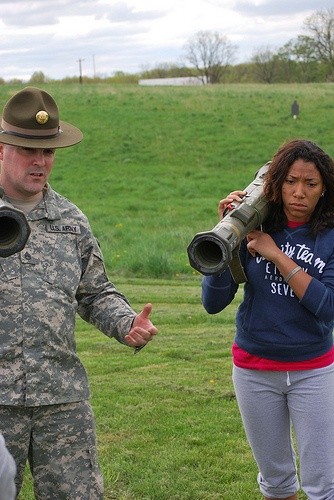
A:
[0,87,83,148]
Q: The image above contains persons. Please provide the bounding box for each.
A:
[199,138,334,500]
[0,433,18,500]
[0,86,159,500]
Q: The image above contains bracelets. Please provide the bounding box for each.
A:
[283,266,303,285]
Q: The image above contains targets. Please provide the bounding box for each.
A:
[291,100,299,119]
